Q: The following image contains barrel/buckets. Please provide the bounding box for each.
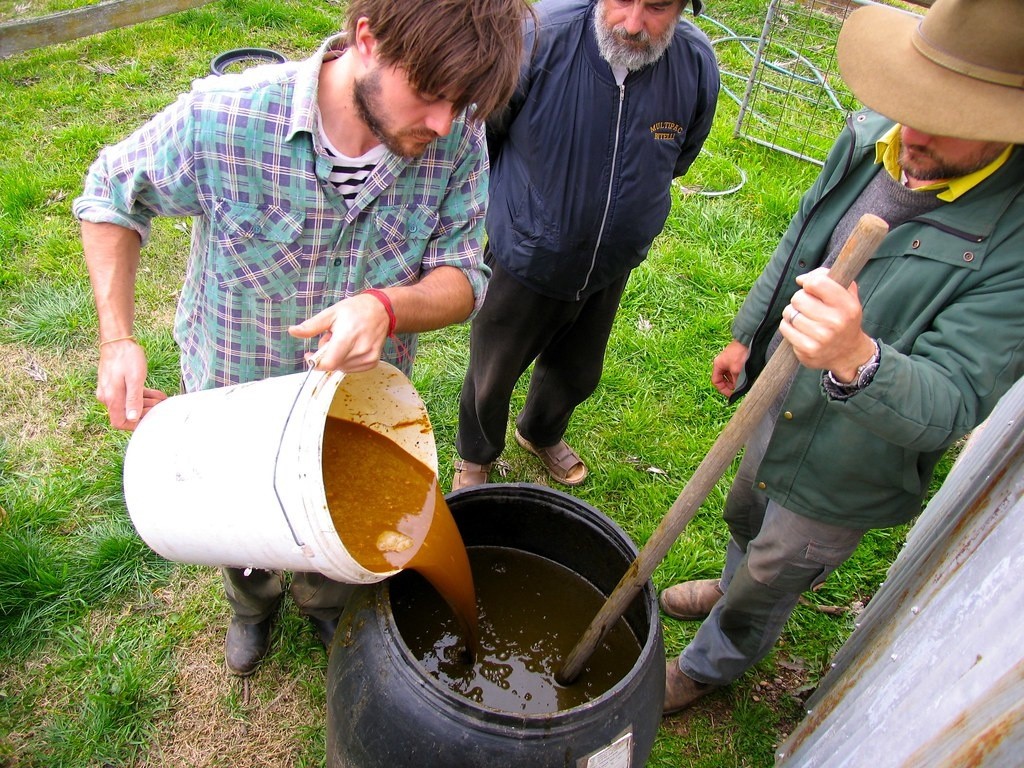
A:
[123,337,437,585]
[325,481,668,768]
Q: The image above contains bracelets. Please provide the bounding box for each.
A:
[98,334,136,349]
[357,289,416,363]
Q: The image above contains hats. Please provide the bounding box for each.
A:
[837,0,1024,143]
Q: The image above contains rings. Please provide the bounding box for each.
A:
[787,310,800,325]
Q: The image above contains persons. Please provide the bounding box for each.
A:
[75,0,541,679]
[659,1,1024,715]
[450,0,719,491]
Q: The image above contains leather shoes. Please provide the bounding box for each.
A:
[663,655,725,717]
[660,578,725,621]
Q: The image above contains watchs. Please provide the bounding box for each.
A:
[828,339,881,393]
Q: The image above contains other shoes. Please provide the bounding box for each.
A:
[310,615,346,656]
[515,427,588,485]
[224,611,272,676]
[452,458,493,493]
